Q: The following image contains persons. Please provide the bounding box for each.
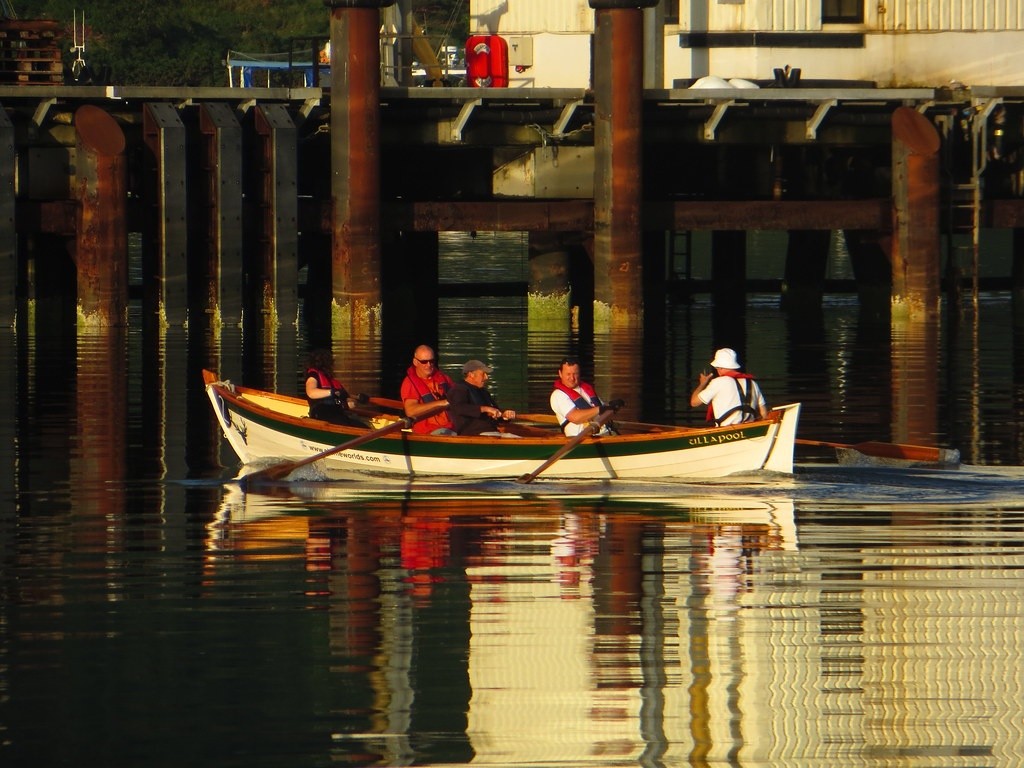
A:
[401,345,457,435]
[550,358,611,437]
[304,352,371,428]
[446,359,516,436]
[691,348,767,427]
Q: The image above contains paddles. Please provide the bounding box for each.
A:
[514,409,614,484]
[795,438,962,462]
[350,395,404,412]
[490,413,559,422]
[238,406,447,484]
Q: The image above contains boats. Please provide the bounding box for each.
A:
[198,366,805,482]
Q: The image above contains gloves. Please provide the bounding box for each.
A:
[608,399,625,408]
[356,393,369,404]
[600,406,618,415]
[335,389,349,400]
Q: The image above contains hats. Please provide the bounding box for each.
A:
[710,348,741,370]
[463,360,493,374]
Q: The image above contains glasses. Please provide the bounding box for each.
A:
[415,358,435,364]
